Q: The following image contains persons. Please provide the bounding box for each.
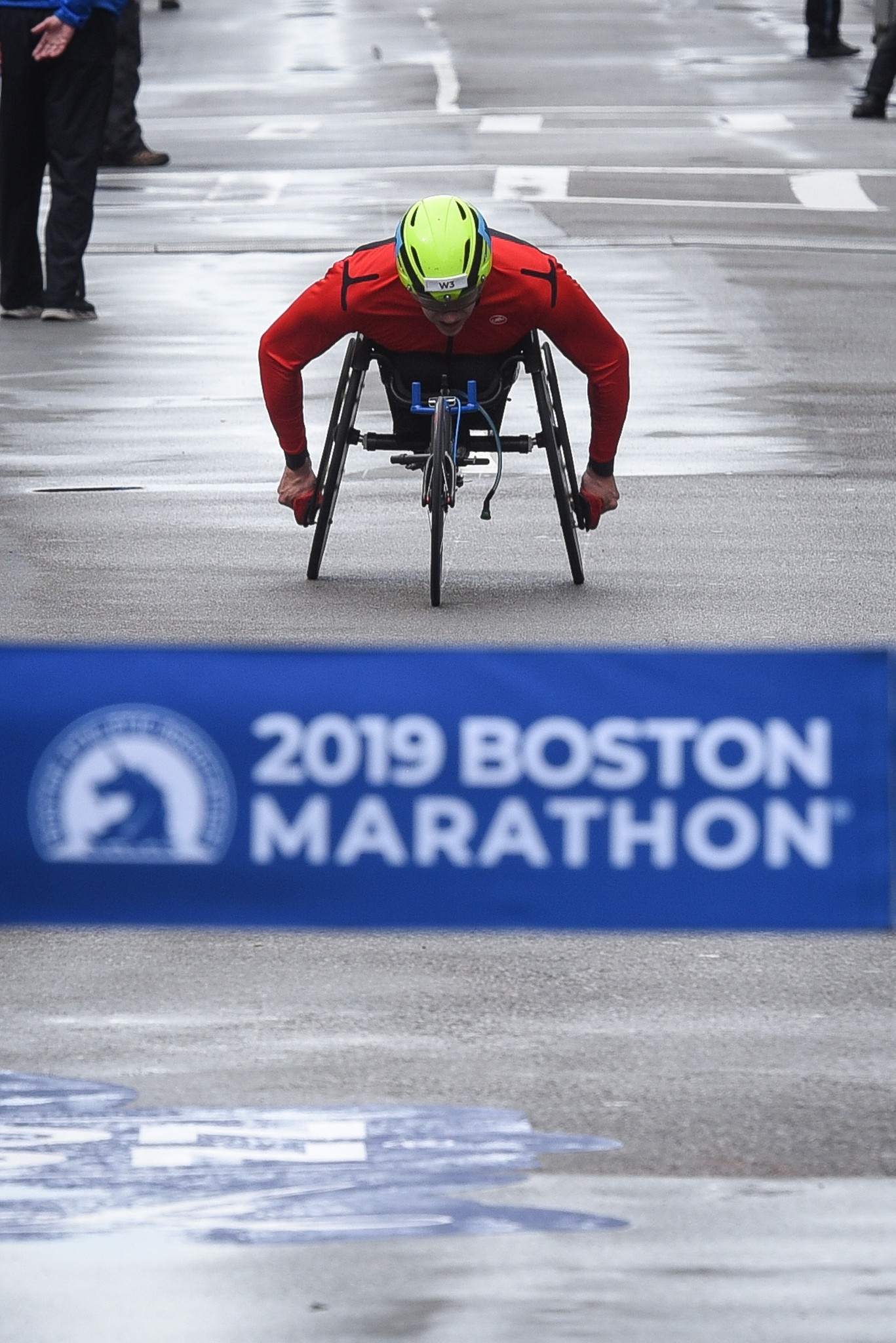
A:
[0,0,128,320]
[806,0,860,57]
[98,0,170,167]
[161,0,179,9]
[259,195,630,531]
[851,0,896,119]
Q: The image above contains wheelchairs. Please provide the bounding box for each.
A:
[298,327,611,607]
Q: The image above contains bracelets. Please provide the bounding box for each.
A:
[284,447,310,470]
[589,458,614,477]
